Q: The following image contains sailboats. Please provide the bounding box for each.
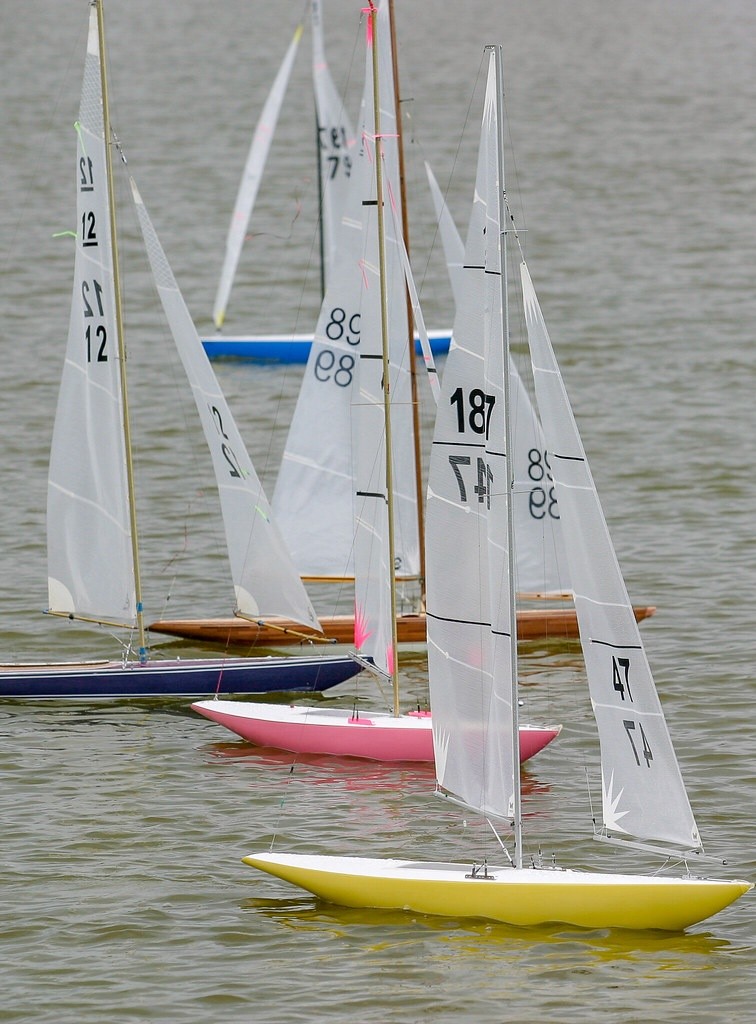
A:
[1,0,373,702]
[141,1,659,656]
[188,1,561,768]
[201,0,455,366]
[240,41,756,936]
